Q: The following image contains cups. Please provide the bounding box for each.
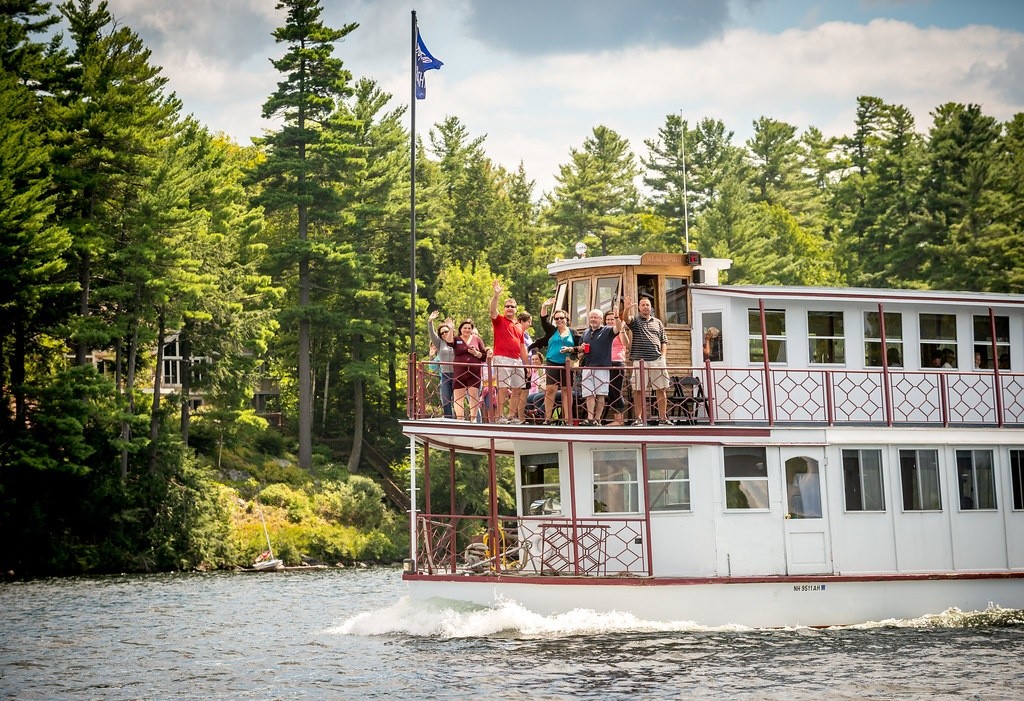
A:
[584,345,590,353]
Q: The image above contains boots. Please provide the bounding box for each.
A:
[607,414,627,427]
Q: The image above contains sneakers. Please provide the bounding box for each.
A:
[657,417,675,426]
[630,416,646,428]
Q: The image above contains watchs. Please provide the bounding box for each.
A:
[568,346,572,353]
[613,316,619,319]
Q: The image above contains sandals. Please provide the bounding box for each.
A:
[578,418,593,426]
[590,417,605,426]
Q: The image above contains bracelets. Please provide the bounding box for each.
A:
[625,328,630,331]
[619,331,625,333]
[574,346,578,352]
[473,351,479,357]
[542,305,547,309]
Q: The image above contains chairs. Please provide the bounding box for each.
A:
[524,375,717,427]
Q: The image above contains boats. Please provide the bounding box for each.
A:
[390,247,1024,633]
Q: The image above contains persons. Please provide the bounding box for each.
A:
[467,326,479,336]
[526,351,549,424]
[886,347,901,367]
[603,309,628,427]
[531,385,575,425]
[489,278,531,425]
[622,295,675,426]
[579,302,622,425]
[539,296,580,427]
[864,311,891,338]
[975,352,982,369]
[623,315,636,347]
[614,298,626,321]
[509,313,537,423]
[480,347,498,425]
[999,353,1010,369]
[445,316,487,424]
[959,496,976,511]
[427,310,459,419]
[607,480,626,513]
[428,340,455,419]
[703,326,713,362]
[940,348,956,368]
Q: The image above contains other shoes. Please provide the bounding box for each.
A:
[496,417,509,425]
[542,419,551,426]
[511,418,527,425]
[562,420,573,425]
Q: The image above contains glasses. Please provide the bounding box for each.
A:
[504,304,517,309]
[607,318,615,321]
[554,317,567,320]
[947,356,955,359]
[438,330,451,335]
